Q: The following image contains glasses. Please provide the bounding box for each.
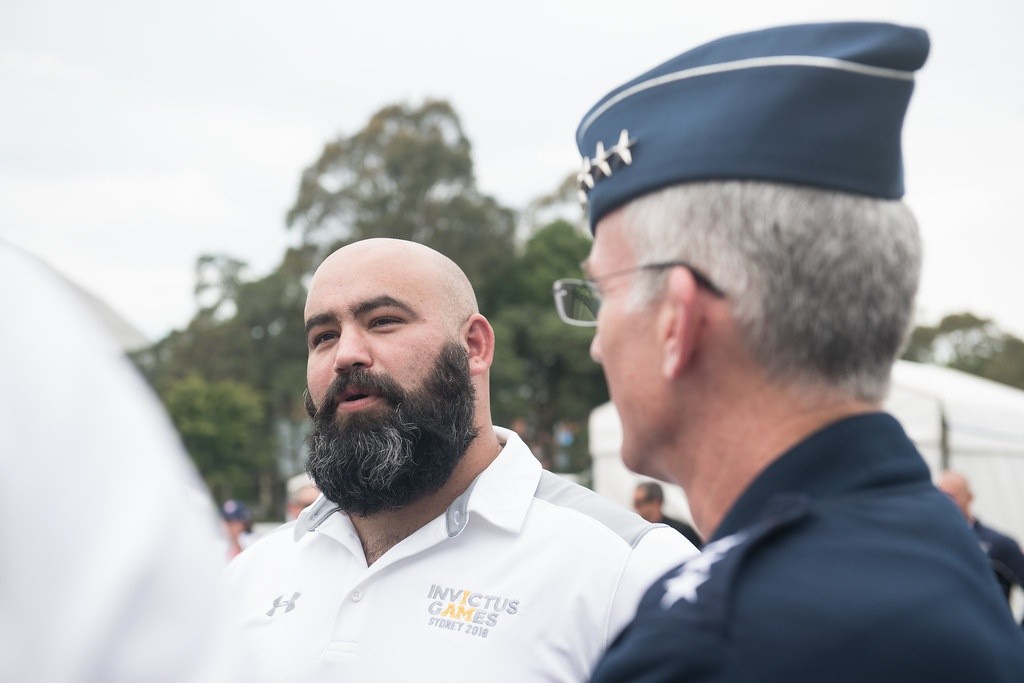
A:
[548,260,728,329]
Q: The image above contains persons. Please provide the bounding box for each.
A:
[936,473,1024,627]
[552,21,1024,683]
[635,483,703,551]
[185,238,702,683]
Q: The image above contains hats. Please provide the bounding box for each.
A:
[575,23,930,238]
